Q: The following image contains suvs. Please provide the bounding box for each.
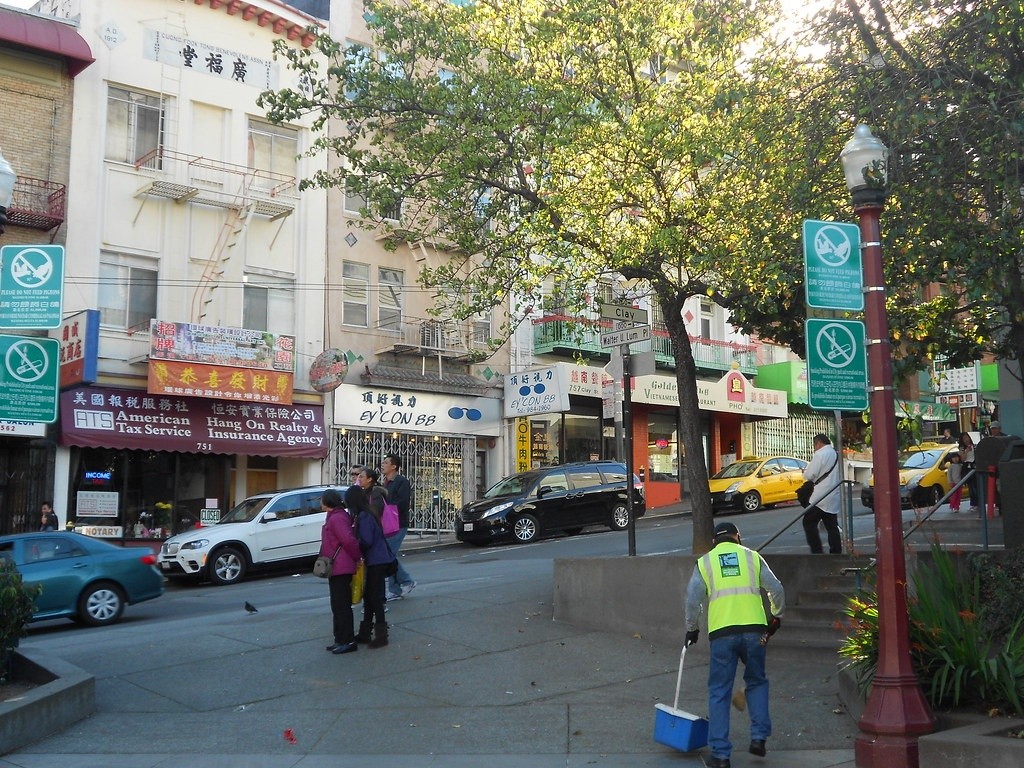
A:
[454,460,648,546]
[154,483,353,586]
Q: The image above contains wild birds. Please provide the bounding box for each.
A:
[244,601,258,614]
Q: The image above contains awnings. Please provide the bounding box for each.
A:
[59,384,326,459]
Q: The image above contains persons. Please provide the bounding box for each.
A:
[39,503,58,532]
[948,452,962,513]
[803,434,842,554]
[939,429,956,444]
[990,421,1007,437]
[684,522,786,768]
[319,454,416,654]
[959,433,978,512]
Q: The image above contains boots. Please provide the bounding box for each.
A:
[369,623,389,648]
[355,621,374,644]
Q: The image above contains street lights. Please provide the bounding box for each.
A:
[837,124,940,768]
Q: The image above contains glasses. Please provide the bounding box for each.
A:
[351,472,359,475]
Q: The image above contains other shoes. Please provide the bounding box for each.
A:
[748,739,766,757]
[705,756,732,768]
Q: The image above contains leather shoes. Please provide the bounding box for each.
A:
[327,642,341,650]
[333,642,357,653]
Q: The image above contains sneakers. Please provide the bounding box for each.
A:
[971,506,979,512]
[401,581,416,595]
[360,606,388,615]
[968,506,975,511]
[387,592,403,601]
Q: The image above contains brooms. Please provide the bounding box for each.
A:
[732,687,746,713]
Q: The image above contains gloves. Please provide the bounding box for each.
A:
[768,615,781,636]
[684,629,700,648]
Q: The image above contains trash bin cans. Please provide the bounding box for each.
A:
[998,439,1024,552]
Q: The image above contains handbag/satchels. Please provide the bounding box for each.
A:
[796,481,814,509]
[381,494,399,538]
[352,564,366,604]
[313,555,333,578]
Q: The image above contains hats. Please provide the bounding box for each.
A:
[713,522,740,537]
[990,420,1002,429]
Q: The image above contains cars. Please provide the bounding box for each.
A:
[0,527,167,627]
[860,443,971,512]
[708,454,811,515]
